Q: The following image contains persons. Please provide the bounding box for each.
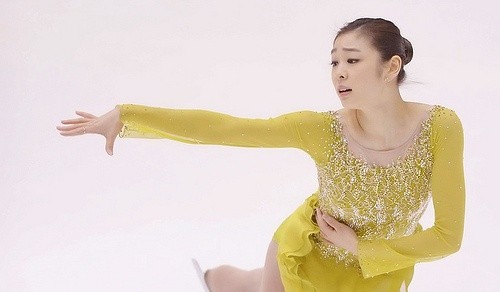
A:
[56,17,466,292]
